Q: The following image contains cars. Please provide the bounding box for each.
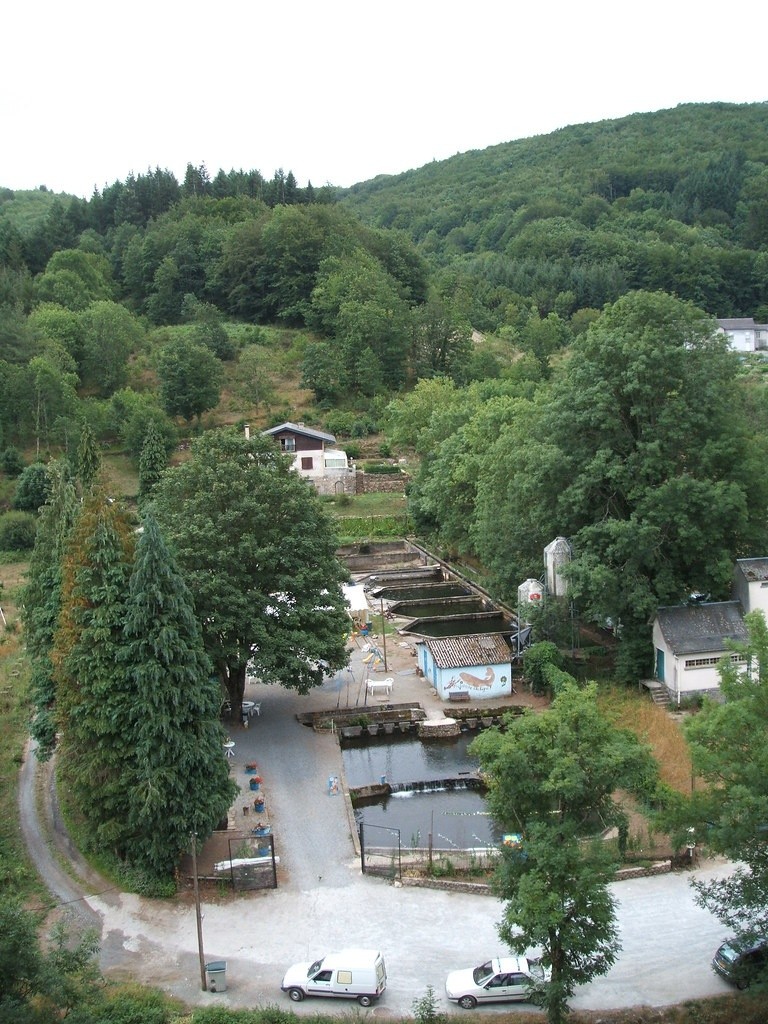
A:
[710,932,768,992]
[281,948,388,1008]
[443,955,554,1012]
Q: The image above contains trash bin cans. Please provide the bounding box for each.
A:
[205,961,227,993]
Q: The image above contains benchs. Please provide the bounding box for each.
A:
[449,691,470,703]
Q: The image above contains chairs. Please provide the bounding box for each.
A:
[224,701,231,716]
[253,701,262,716]
[384,678,395,692]
[365,679,377,693]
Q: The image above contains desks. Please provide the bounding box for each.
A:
[242,702,255,716]
[368,681,392,696]
